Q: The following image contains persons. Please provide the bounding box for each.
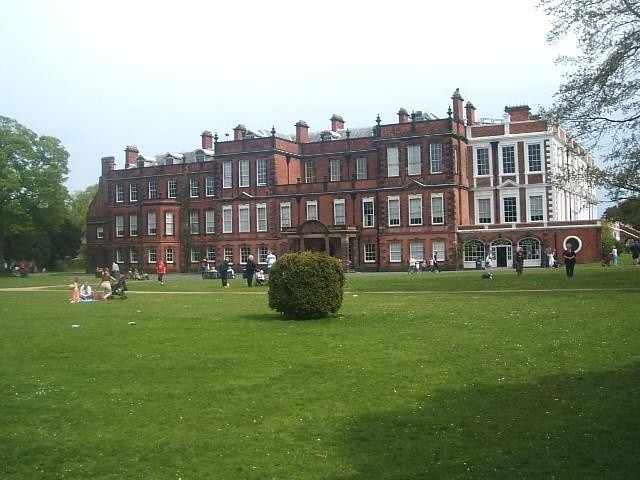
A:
[10,258,29,278]
[201,250,276,288]
[486,251,491,272]
[409,251,441,274]
[609,246,617,266]
[545,242,576,278]
[516,247,523,280]
[630,241,640,265]
[68,260,166,302]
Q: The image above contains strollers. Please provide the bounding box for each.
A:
[108,276,127,301]
[255,269,264,286]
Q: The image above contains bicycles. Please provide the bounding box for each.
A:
[407,260,439,278]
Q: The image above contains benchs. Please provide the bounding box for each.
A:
[202,271,234,279]
